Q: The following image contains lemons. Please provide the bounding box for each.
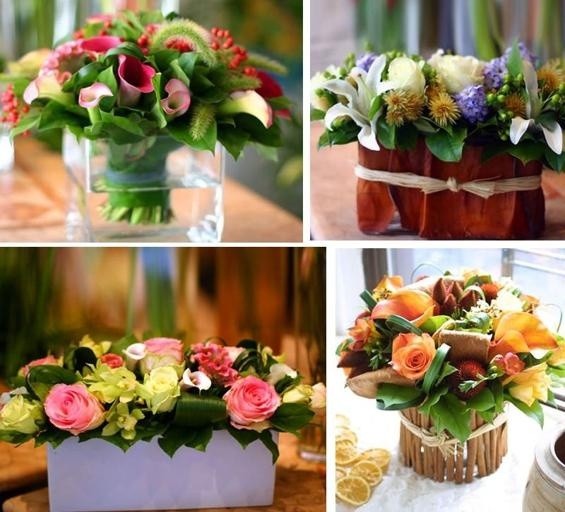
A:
[337,413,391,509]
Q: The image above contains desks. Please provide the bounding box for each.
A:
[3,133,301,244]
[310,114,565,237]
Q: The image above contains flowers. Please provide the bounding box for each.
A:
[0,335,317,453]
[309,39,565,172]
[0,10,294,227]
[335,272,565,431]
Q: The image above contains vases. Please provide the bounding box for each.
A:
[343,121,549,240]
[391,400,513,486]
[60,122,226,245]
[46,430,281,512]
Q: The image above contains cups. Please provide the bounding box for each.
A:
[296,410,326,463]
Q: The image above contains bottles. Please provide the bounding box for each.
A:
[521,423,565,512]
